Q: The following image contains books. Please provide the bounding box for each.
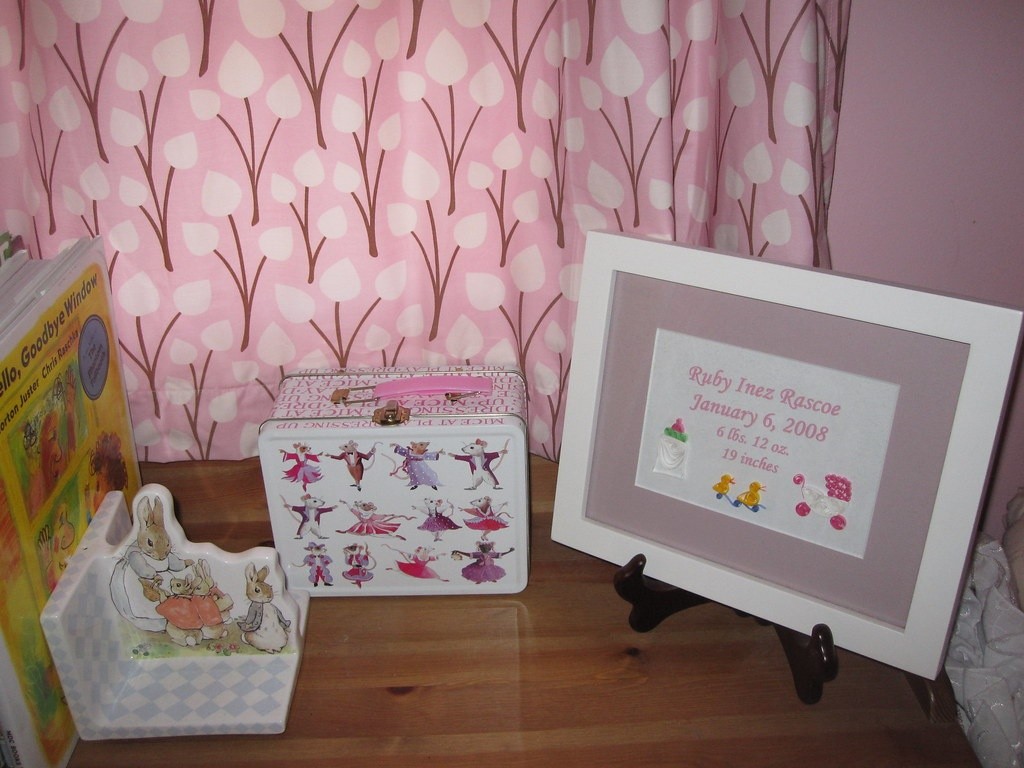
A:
[0,230,143,768]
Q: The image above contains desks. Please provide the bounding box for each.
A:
[56,447,981,767]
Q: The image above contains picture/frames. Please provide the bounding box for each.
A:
[551,228,1024,680]
